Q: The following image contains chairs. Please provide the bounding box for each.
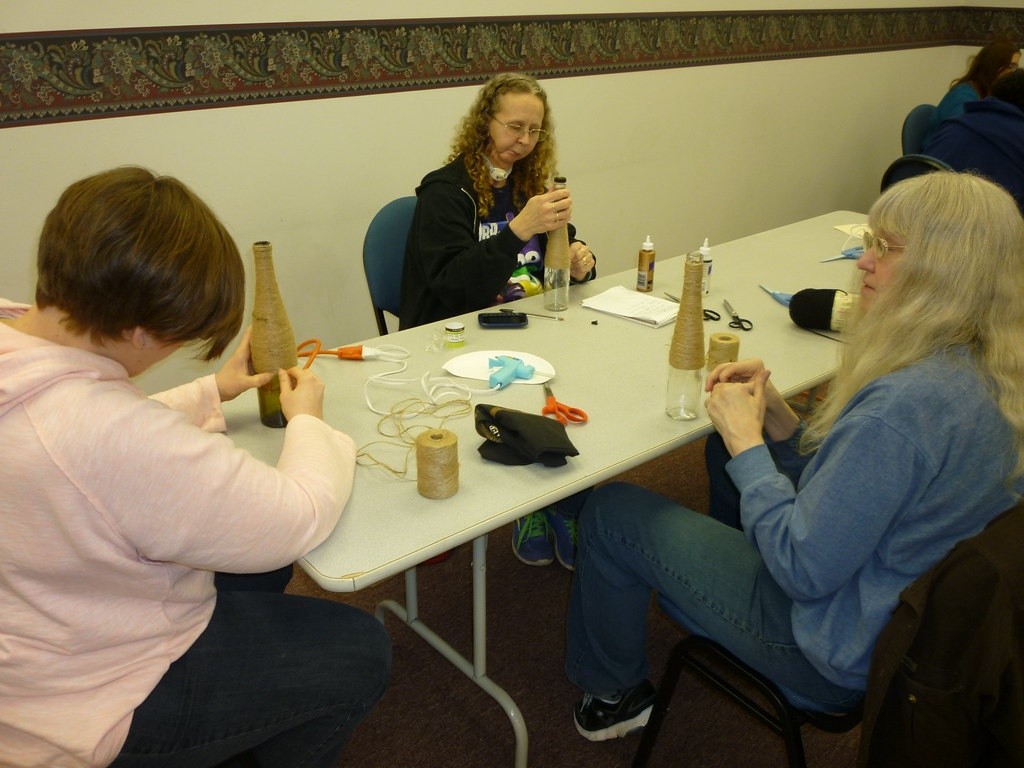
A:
[630,508,1024,768]
[880,102,960,204]
[361,194,423,336]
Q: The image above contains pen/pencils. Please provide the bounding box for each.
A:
[499,309,564,321]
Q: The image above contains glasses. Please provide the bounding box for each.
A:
[862,230,910,257]
[487,112,549,142]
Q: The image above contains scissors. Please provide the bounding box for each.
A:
[759,284,794,305]
[819,246,864,263]
[540,380,588,426]
[663,292,721,321]
[723,300,753,331]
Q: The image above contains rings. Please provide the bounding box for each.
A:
[583,256,589,264]
[553,203,558,212]
[557,213,559,222]
[581,249,587,256]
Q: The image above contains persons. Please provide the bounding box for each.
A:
[1,164,393,768]
[564,171,1024,741]
[399,72,598,570]
[924,39,1024,218]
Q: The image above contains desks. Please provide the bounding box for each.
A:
[207,209,883,768]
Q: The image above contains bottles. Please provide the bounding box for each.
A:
[248,241,299,427]
[699,238,713,296]
[545,176,571,311]
[637,235,656,291]
[665,252,704,421]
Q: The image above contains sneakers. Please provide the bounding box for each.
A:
[574,681,661,740]
[545,506,581,571]
[512,508,555,565]
